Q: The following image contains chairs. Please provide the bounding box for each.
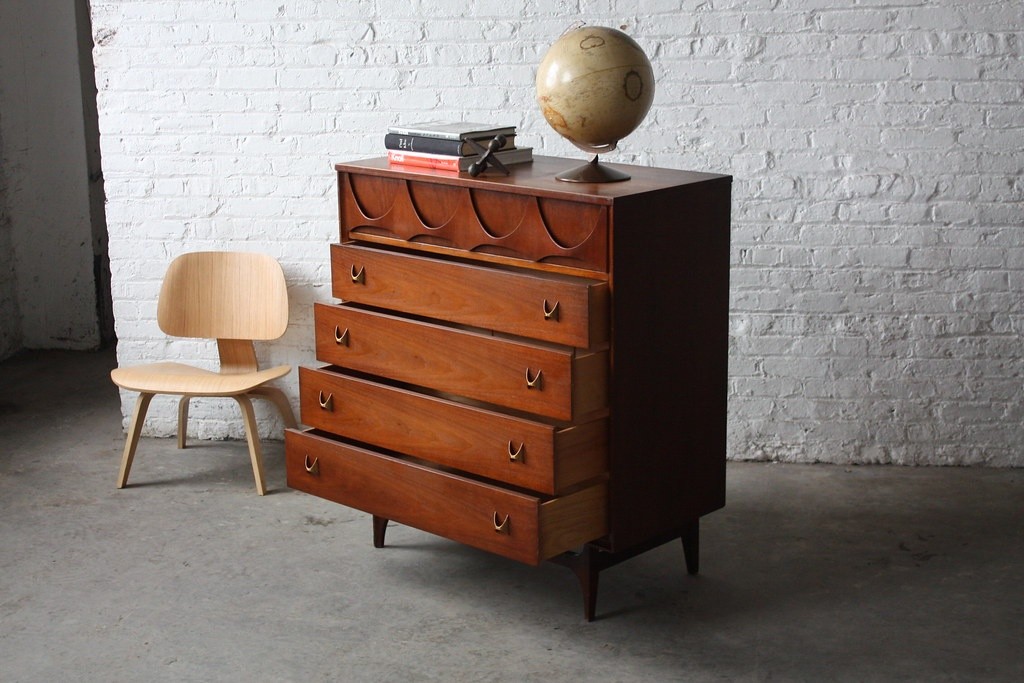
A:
[111,252,298,496]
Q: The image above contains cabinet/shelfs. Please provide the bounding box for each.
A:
[284,154,734,622]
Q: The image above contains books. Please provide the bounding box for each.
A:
[385,119,534,173]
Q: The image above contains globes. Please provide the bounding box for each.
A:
[536,27,655,184]
